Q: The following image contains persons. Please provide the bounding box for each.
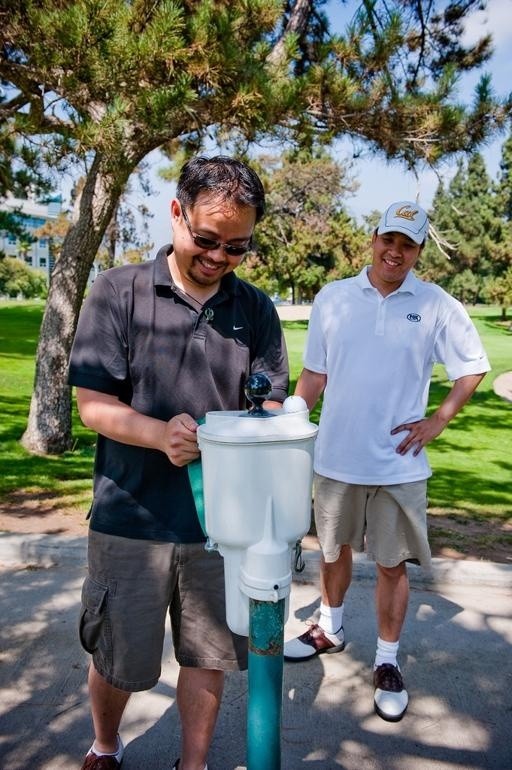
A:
[64,153,289,770]
[283,197,495,723]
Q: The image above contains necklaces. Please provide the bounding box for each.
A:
[177,285,208,309]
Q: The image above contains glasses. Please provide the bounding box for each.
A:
[178,200,249,257]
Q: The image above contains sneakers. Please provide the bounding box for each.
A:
[82,730,124,769]
[372,661,408,722]
[284,627,346,662]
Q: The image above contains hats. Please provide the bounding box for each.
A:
[373,201,429,249]
